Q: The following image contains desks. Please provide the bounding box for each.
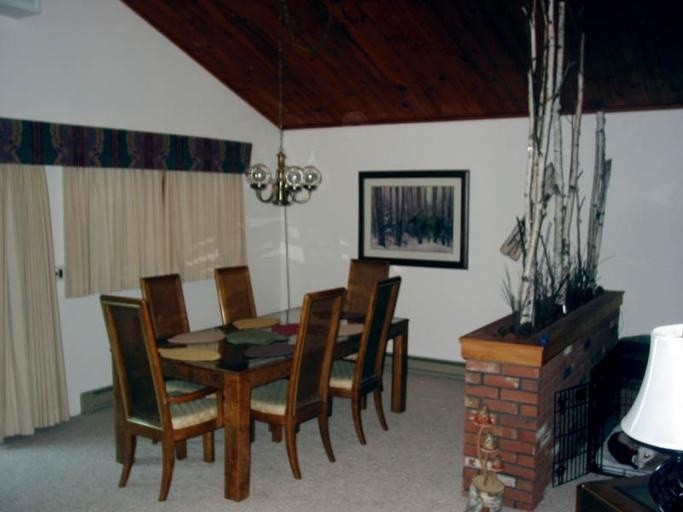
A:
[155,304,410,503]
[571,475,657,512]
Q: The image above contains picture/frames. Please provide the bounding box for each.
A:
[355,167,470,273]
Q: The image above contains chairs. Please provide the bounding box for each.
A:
[249,285,348,480]
[326,275,401,450]
[213,266,261,325]
[338,257,390,363]
[138,270,221,464]
[93,291,227,503]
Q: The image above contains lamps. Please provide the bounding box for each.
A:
[241,0,323,208]
[613,320,682,512]
